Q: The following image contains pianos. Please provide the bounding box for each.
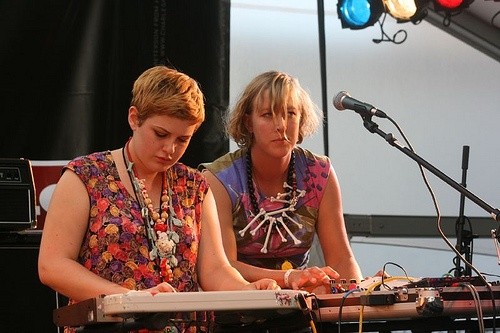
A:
[53,290,313,328]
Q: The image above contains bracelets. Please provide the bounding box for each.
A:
[284,269,294,289]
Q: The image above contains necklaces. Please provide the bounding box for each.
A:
[239,142,307,253]
[122,134,181,285]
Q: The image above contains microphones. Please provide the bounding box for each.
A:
[333,90,387,118]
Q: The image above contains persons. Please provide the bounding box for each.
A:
[37,66,288,333]
[199,71,398,333]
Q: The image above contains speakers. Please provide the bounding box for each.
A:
[0,232,65,333]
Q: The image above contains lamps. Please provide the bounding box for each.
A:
[337,0,475,29]
